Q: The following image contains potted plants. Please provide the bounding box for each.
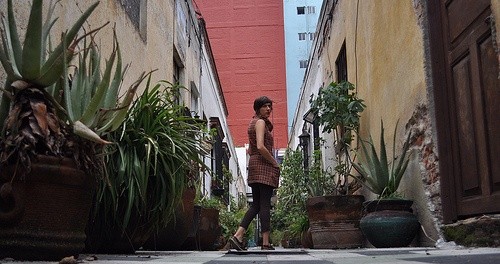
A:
[0,0,421,261]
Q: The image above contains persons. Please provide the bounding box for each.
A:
[228,96,282,252]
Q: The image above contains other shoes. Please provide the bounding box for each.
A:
[229,236,247,251]
[261,245,275,250]
